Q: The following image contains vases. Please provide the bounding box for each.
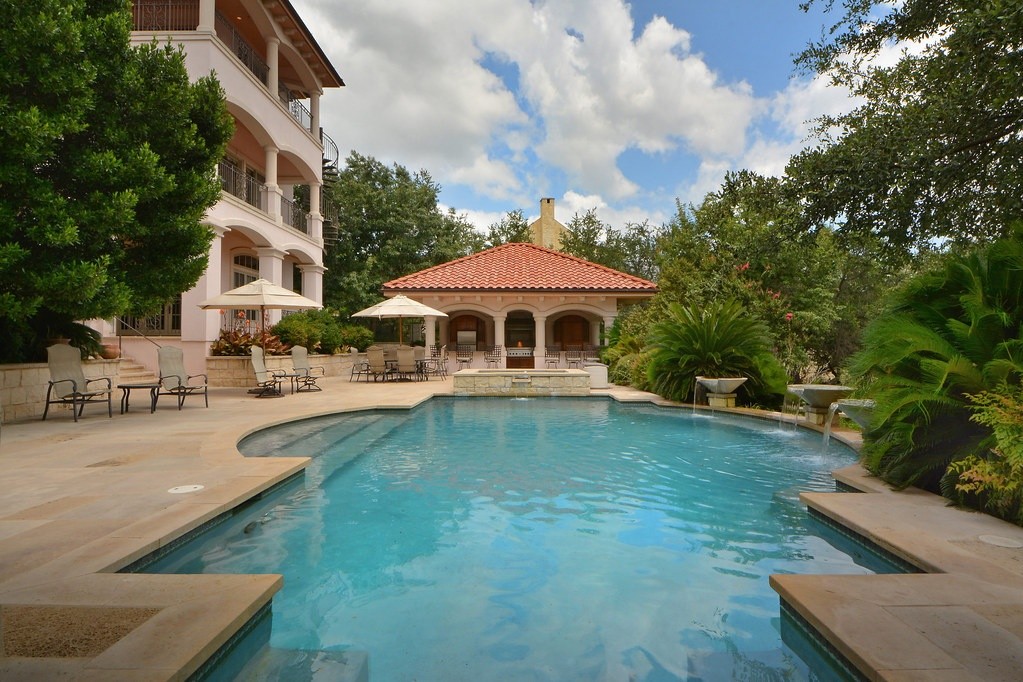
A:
[100,344,120,359]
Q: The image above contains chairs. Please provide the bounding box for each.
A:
[290,345,325,392]
[350,344,450,382]
[42,345,112,421]
[251,345,287,398]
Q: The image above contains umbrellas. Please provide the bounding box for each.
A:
[351,294,449,347]
[197,278,324,366]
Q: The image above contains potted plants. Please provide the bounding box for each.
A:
[49,334,72,344]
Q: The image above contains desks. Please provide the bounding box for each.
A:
[117,382,163,415]
[272,373,300,395]
[364,357,447,382]
[156,346,208,411]
